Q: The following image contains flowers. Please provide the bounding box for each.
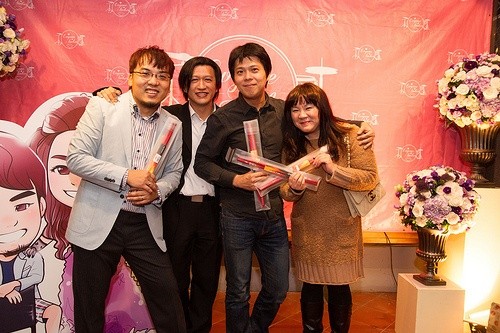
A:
[0,1,31,78]
[394,165,480,234]
[438,52,500,128]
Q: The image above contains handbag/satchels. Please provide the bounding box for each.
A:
[344,124,387,218]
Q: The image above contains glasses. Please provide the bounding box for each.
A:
[130,69,172,82]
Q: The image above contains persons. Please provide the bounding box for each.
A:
[92,56,223,333]
[65,45,185,333]
[192,43,375,333]
[279,81,380,333]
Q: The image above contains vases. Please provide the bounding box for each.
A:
[453,120,500,188]
[413,227,450,286]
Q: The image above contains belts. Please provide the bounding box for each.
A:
[180,194,216,203]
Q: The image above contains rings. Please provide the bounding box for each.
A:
[148,182,151,186]
[141,196,144,200]
